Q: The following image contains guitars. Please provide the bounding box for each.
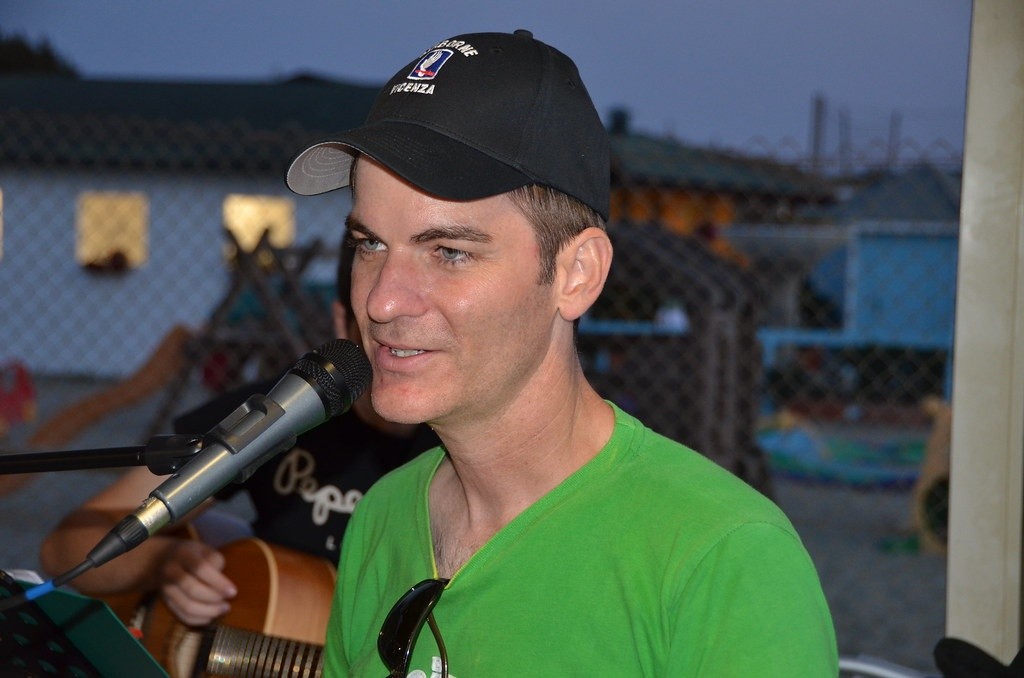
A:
[96,519,339,678]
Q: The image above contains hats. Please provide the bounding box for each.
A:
[283,31,611,223]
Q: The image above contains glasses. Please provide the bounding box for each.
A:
[379,579,448,677]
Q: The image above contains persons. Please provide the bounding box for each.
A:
[287,28,841,678]
[41,227,440,624]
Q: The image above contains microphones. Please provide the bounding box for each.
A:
[89,338,373,569]
